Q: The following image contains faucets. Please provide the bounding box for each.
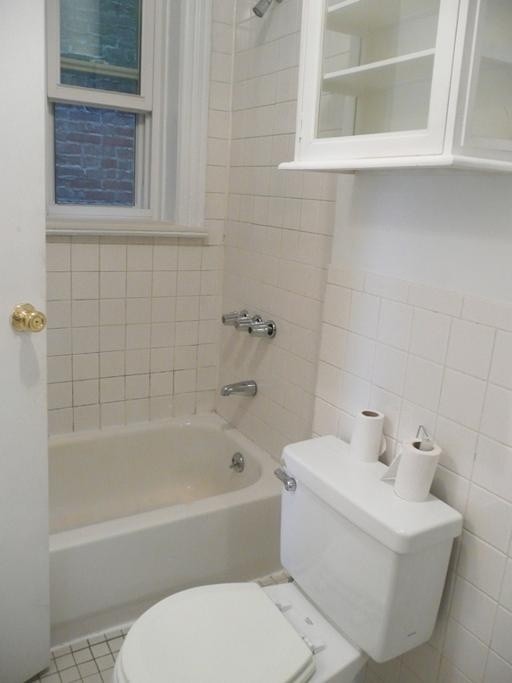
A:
[221,381,256,397]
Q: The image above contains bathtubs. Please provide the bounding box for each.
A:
[48,414,286,650]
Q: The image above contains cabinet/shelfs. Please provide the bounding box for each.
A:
[277,0,512,178]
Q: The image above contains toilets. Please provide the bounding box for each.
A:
[114,434,463,683]
